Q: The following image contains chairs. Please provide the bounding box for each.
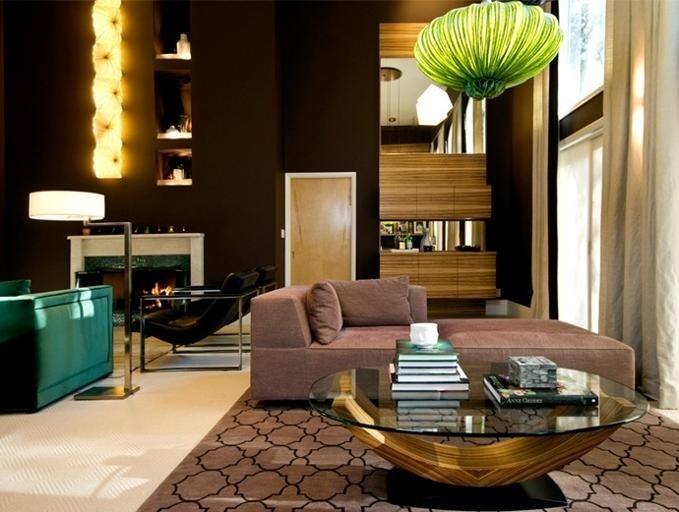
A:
[0,278,114,414]
[173,265,278,354]
[139,270,259,372]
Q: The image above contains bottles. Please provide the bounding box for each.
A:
[176,33,191,59]
[394,227,405,250]
[404,233,413,249]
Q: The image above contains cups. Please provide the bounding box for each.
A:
[409,322,439,349]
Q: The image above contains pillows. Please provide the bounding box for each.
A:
[327,275,415,328]
[306,280,344,345]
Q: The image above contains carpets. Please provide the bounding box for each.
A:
[136,386,679,512]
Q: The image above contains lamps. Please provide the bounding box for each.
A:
[28,191,140,400]
[413,0,564,100]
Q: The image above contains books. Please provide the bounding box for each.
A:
[389,339,470,408]
[484,374,597,409]
[395,408,461,426]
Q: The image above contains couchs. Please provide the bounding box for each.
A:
[249,275,636,404]
